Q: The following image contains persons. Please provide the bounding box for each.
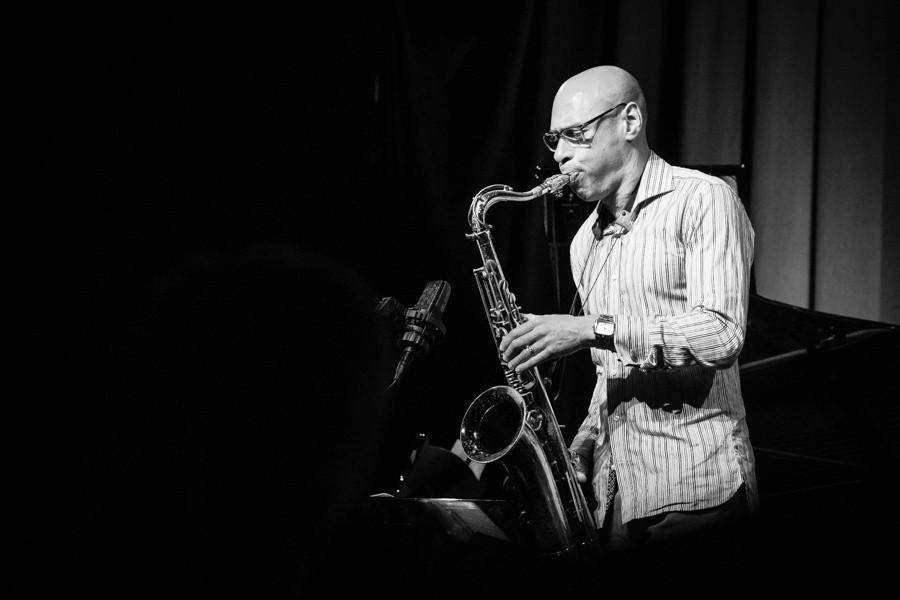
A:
[500,66,771,600]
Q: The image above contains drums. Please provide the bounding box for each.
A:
[366,496,520,570]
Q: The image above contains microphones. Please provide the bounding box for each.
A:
[394,278,452,385]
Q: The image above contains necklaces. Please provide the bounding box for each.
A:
[553,222,624,403]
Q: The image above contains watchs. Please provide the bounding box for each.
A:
[592,314,615,349]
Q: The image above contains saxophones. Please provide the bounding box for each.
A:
[459,171,606,578]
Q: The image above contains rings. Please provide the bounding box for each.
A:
[527,345,535,356]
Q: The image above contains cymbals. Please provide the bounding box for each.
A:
[395,442,481,498]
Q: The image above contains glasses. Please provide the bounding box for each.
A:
[542,102,629,152]
